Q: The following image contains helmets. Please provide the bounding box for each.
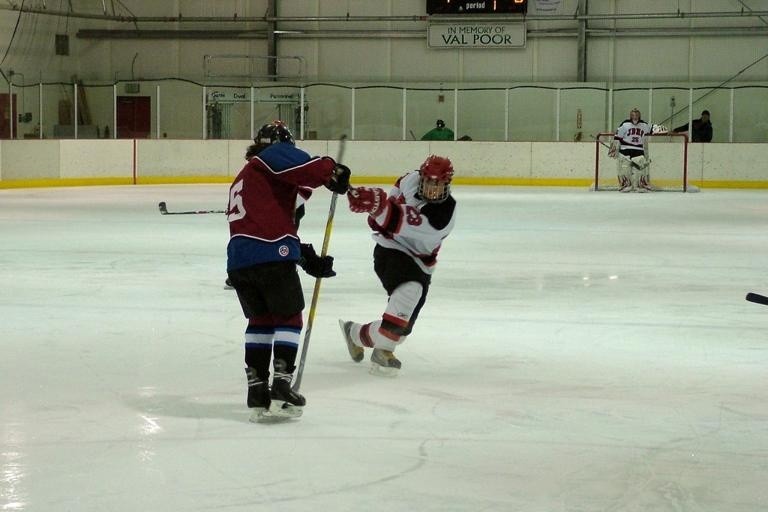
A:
[254,120,295,148]
[418,154,454,204]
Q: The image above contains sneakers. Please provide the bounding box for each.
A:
[342,321,365,361]
[370,348,401,368]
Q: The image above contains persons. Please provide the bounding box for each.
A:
[608,107,669,192]
[342,155,457,369]
[224,185,313,290]
[420,118,453,140]
[225,119,351,408]
[673,109,714,142]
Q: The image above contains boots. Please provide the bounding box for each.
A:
[271,359,305,406]
[245,367,271,409]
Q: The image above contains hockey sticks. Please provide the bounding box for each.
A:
[282,136,346,421]
[590,135,651,170]
[159,203,228,215]
[746,293,768,305]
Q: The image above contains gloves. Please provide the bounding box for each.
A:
[328,164,352,194]
[297,245,335,278]
[348,187,384,214]
